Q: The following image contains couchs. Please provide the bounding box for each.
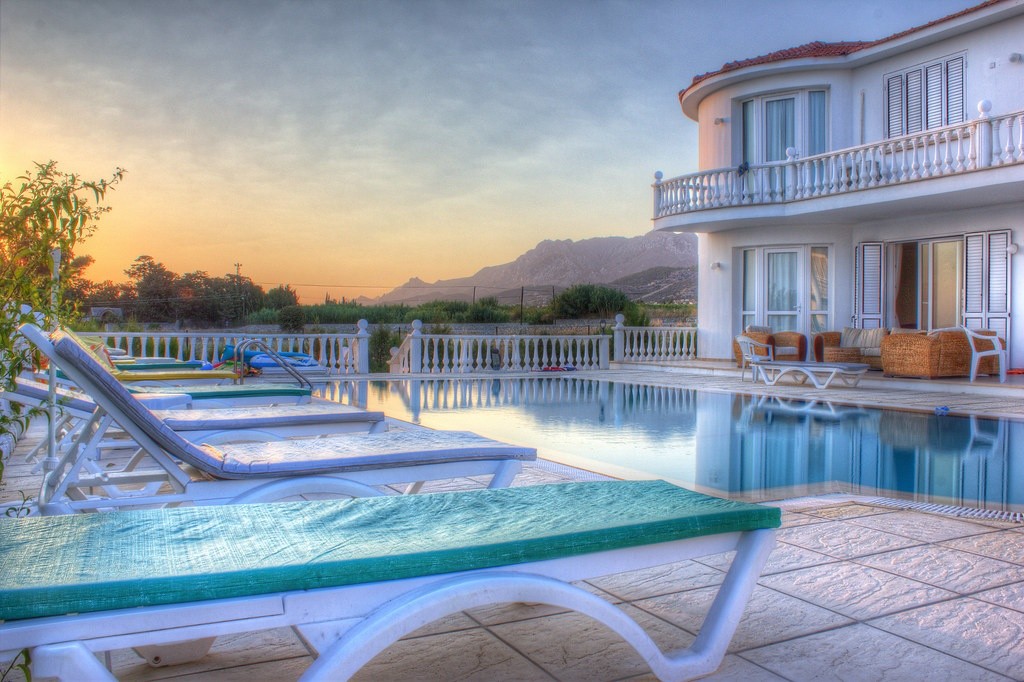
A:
[733,331,808,369]
[813,332,1007,381]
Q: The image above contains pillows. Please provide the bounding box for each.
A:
[890,327,927,334]
[746,325,773,334]
[840,327,889,348]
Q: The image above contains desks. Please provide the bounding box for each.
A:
[823,346,861,363]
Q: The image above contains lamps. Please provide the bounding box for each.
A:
[714,116,723,125]
[1007,243,1020,253]
[1008,52,1022,62]
[710,261,722,270]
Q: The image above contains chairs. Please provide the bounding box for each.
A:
[110,355,175,364]
[37,327,537,515]
[44,362,202,390]
[959,324,1007,384]
[0,377,193,474]
[17,321,389,498]
[735,334,774,384]
[0,477,782,682]
[53,323,238,387]
[749,359,870,390]
[33,362,312,450]
